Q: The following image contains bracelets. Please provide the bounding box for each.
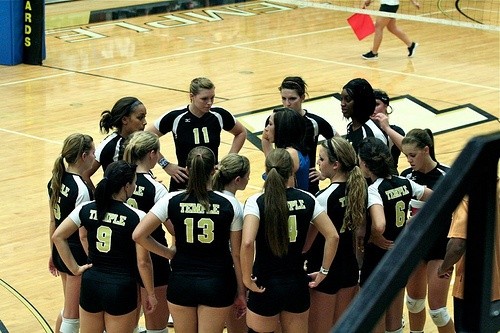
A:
[157,157,169,169]
[319,266,329,276]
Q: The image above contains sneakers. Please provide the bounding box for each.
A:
[361,51,379,60]
[407,42,418,57]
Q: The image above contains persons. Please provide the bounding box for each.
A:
[362,0,420,59]
[47,73,500,333]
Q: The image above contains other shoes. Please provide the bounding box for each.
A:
[167,314,174,328]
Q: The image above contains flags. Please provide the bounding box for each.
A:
[347,9,376,41]
